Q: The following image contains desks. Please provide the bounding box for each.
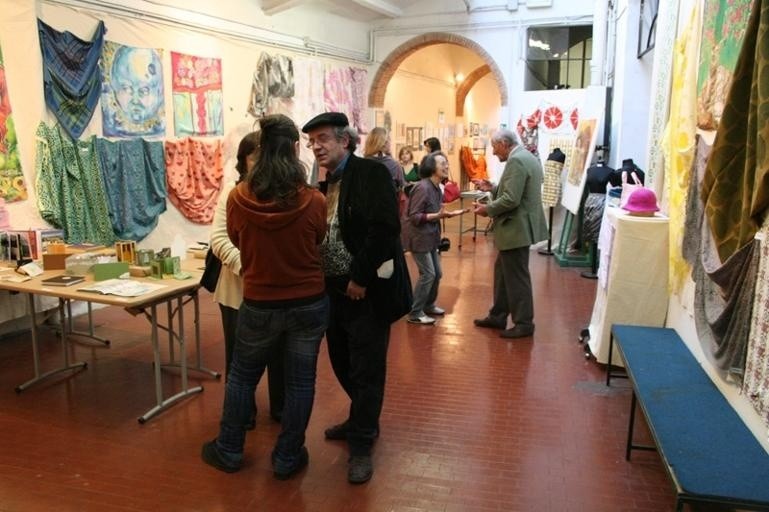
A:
[152,245,221,381]
[458,190,486,251]
[5,245,118,346]
[24,260,204,424]
[1,255,86,390]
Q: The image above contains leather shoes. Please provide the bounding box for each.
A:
[474,317,506,330]
[500,324,533,338]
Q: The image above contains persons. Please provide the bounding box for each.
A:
[543,148,565,207]
[302,111,413,486]
[396,146,420,194]
[423,137,449,186]
[471,128,550,339]
[203,113,328,477]
[583,159,613,241]
[598,159,644,250]
[401,151,469,324]
[364,128,403,196]
[208,131,288,431]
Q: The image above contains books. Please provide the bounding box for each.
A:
[41,276,85,286]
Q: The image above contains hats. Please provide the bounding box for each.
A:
[622,188,660,213]
[303,112,349,133]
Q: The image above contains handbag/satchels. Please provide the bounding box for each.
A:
[201,245,220,292]
[442,181,460,203]
[620,183,642,209]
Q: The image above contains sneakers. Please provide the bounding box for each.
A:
[407,307,445,324]
[274,446,309,480]
[325,422,379,483]
[201,441,240,472]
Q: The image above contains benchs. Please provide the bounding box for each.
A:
[606,324,769,512]
